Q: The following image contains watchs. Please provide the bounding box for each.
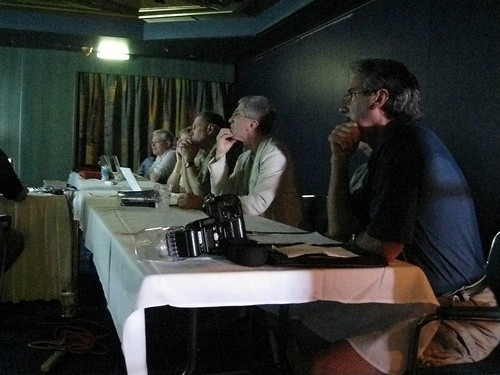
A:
[185,161,194,168]
[348,229,362,245]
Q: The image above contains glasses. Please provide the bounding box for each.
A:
[233,109,255,119]
[342,89,375,105]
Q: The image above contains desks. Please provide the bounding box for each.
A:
[0,186,75,317]
[68,174,440,375]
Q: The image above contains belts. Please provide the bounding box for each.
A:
[447,275,489,303]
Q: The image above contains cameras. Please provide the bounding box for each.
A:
[165,194,248,262]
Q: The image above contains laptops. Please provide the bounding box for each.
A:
[100,154,155,191]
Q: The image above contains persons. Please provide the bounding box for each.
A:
[0,147,31,274]
[177,110,237,203]
[206,93,304,235]
[146,128,183,186]
[289,56,500,375]
[137,152,158,180]
[165,126,207,195]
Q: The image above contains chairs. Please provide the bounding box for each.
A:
[404,231,500,375]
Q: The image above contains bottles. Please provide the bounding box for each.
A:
[61,282,73,317]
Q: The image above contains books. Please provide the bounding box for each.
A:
[79,170,101,179]
